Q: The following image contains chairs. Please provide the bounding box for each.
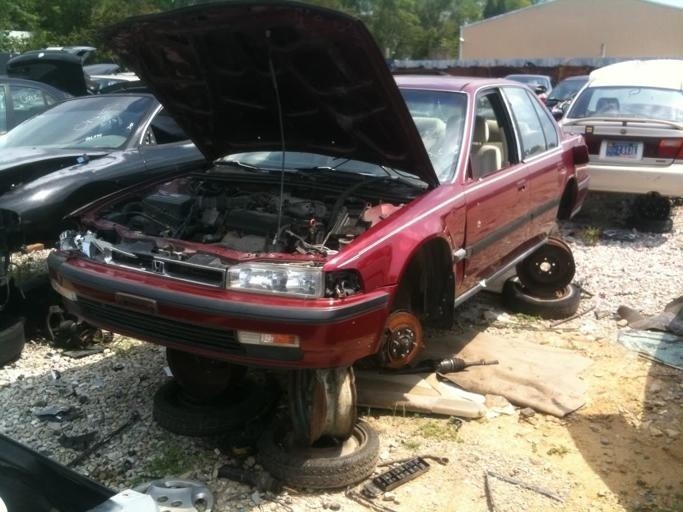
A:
[412,117,508,180]
